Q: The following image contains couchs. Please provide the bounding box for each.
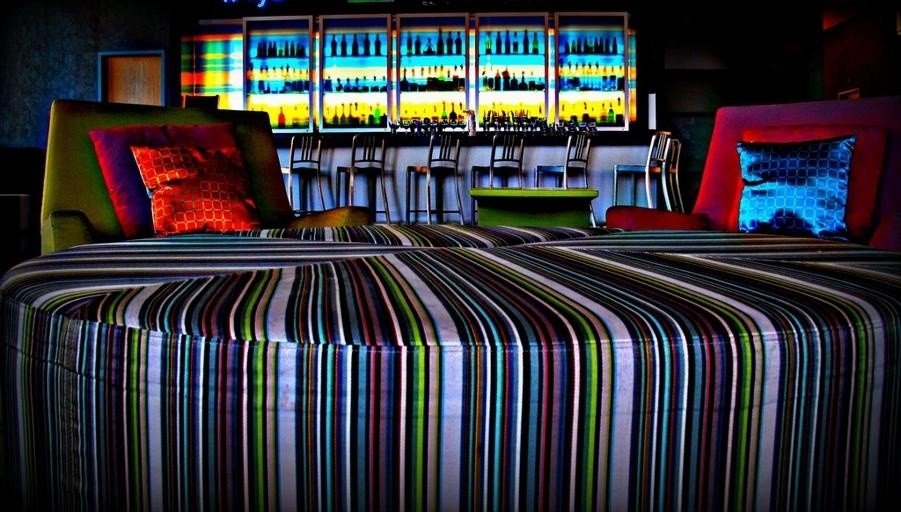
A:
[39,99,375,251]
[605,96,898,252]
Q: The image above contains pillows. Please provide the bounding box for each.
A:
[737,137,855,244]
[130,146,262,238]
[88,123,247,243]
[727,127,885,233]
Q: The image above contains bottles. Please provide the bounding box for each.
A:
[252,27,626,128]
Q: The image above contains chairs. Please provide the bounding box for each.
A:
[406,131,465,225]
[335,133,390,227]
[613,128,671,210]
[471,134,525,224]
[665,139,686,215]
[538,131,597,228]
[280,133,326,213]
